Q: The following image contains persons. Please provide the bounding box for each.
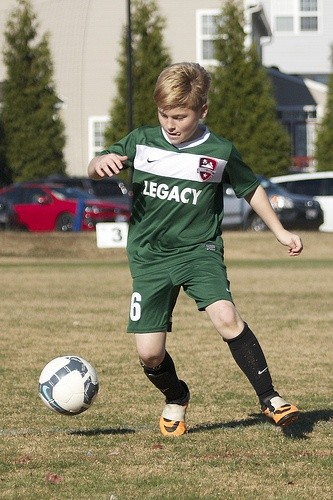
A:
[89,62,304,438]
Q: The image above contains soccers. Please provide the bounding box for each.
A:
[38,354,98,415]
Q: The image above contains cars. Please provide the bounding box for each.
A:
[221,176,324,235]
[0,173,131,233]
[269,169,333,233]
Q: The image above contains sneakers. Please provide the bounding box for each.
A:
[158,379,191,437]
[259,395,301,426]
